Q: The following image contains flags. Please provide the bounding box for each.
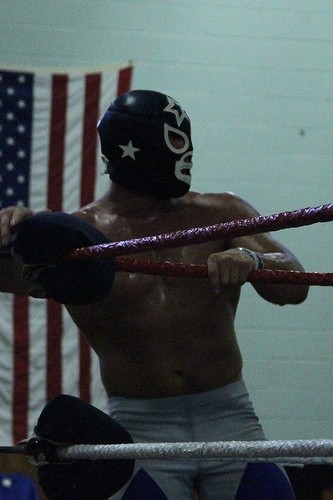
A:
[1,61,138,453]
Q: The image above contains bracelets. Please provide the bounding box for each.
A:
[250,250,265,273]
[236,246,252,255]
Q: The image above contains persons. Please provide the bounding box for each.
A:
[1,88,314,499]
